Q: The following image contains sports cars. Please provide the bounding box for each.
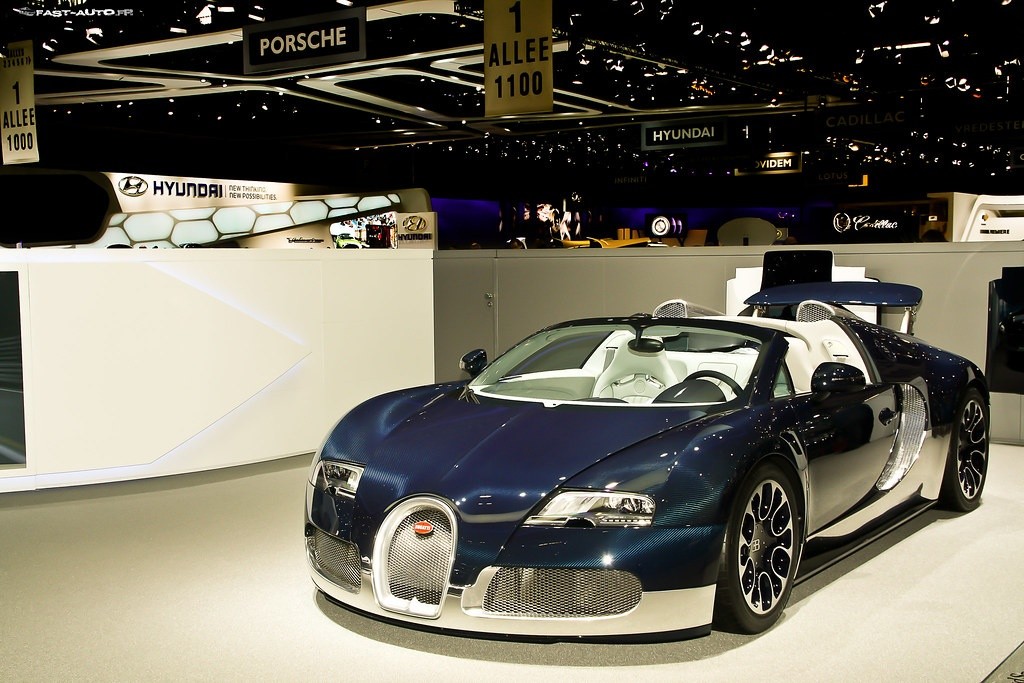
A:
[304,282,991,636]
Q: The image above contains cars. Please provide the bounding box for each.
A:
[336,233,362,248]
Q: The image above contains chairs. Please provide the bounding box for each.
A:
[589,336,680,403]
[732,337,814,402]
